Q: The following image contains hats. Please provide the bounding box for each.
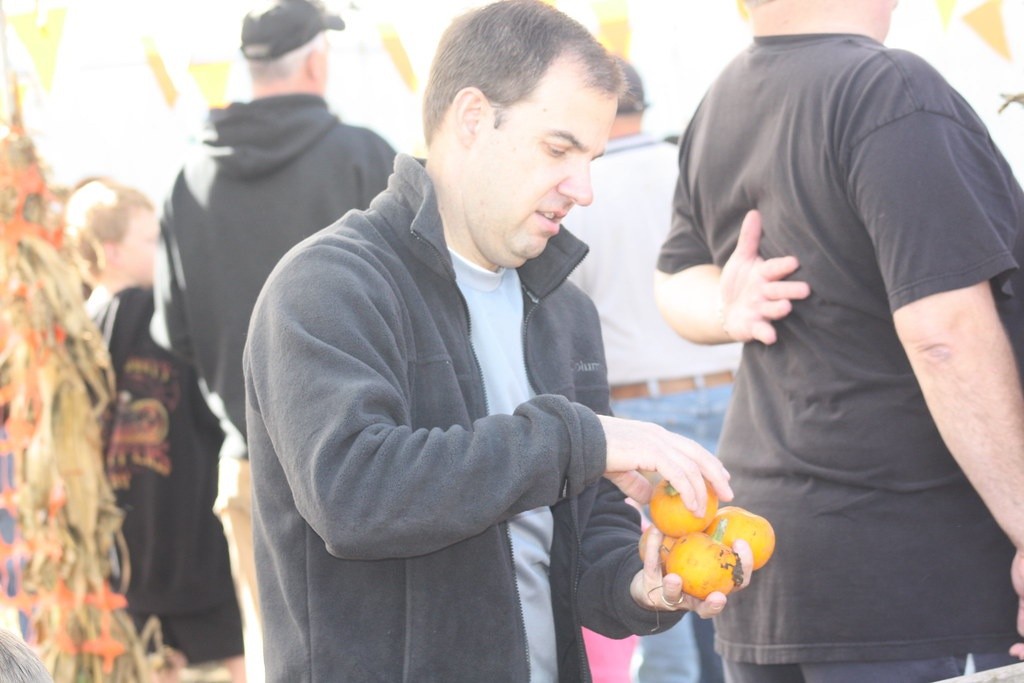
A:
[239,0,345,58]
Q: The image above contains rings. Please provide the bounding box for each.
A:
[661,590,685,606]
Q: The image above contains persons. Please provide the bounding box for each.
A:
[55,174,259,683]
[237,0,758,683]
[151,0,399,683]
[558,50,748,681]
[644,0,1024,681]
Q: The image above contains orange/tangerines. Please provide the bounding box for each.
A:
[637,477,777,602]
